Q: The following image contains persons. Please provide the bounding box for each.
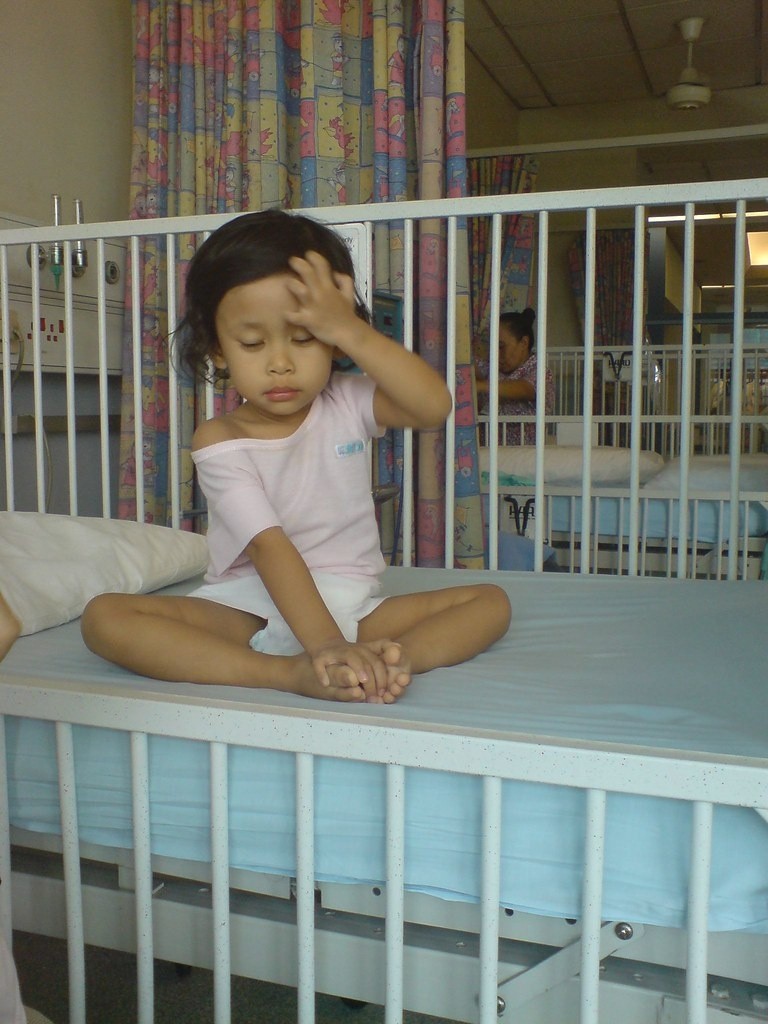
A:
[476,308,554,445]
[0,592,30,1024]
[81,209,512,705]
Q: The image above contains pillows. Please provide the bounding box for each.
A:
[0,511,213,637]
[479,444,664,486]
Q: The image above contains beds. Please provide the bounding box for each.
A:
[0,186,768,1024]
[480,341,768,578]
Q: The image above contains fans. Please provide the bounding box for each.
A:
[666,15,719,112]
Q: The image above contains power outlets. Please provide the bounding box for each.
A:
[0,309,20,353]
